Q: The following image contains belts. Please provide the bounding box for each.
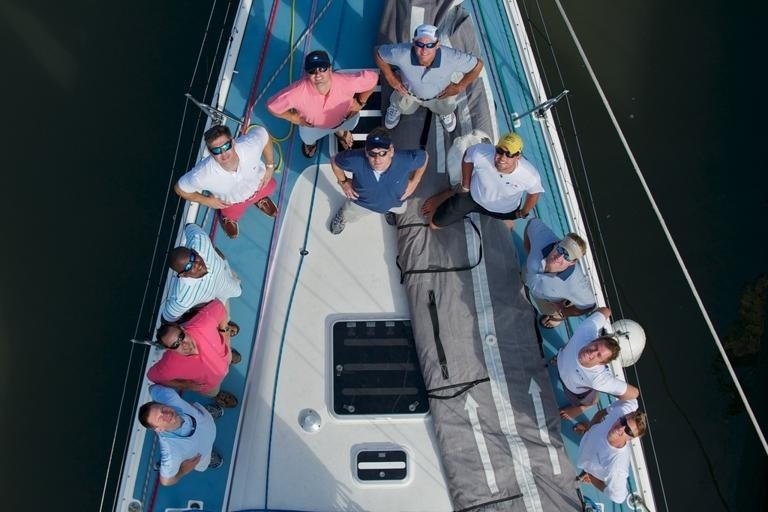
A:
[408,93,437,101]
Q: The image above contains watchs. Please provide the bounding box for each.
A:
[338,176,348,185]
[218,327,229,332]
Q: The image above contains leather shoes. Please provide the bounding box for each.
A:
[222,218,238,238]
[255,197,277,216]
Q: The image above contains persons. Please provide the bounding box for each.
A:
[572,397,649,506]
[330,133,433,235]
[173,124,278,240]
[548,307,641,421]
[376,22,485,135]
[136,384,224,487]
[521,214,596,333]
[268,49,379,159]
[146,298,242,410]
[419,133,548,229]
[159,222,243,323]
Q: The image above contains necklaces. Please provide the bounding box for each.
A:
[222,156,237,172]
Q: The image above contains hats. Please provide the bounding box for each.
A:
[366,129,391,150]
[559,235,583,259]
[305,50,329,70]
[497,133,523,154]
[414,24,438,41]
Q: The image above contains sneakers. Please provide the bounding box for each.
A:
[439,112,456,133]
[385,104,401,129]
[384,212,396,225]
[212,321,241,471]
[330,214,345,233]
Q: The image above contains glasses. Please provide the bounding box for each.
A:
[621,415,634,436]
[556,246,571,260]
[177,250,195,278]
[496,146,514,158]
[415,41,437,47]
[181,413,196,437]
[209,139,232,155]
[307,66,327,74]
[167,331,184,349]
[368,151,387,157]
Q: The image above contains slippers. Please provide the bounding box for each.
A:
[302,143,317,157]
[334,131,353,150]
[540,315,563,328]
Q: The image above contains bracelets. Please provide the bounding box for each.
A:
[604,409,608,415]
[265,163,274,169]
[559,306,565,318]
[519,211,529,218]
[461,186,470,193]
[356,96,367,107]
